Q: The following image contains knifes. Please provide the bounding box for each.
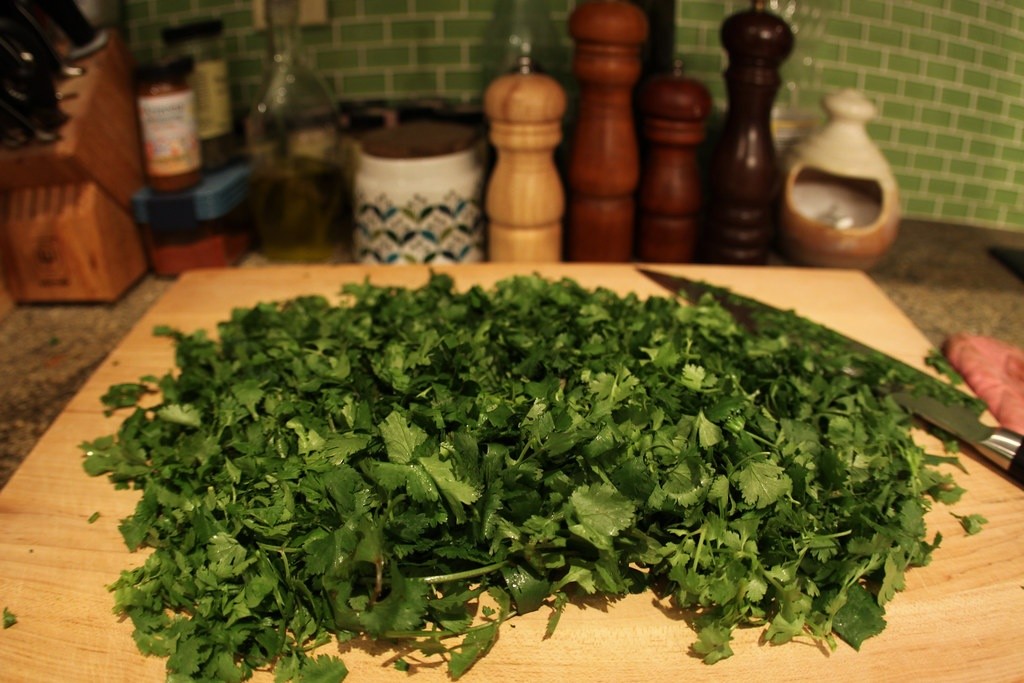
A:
[638,266,1024,488]
[0,0,99,148]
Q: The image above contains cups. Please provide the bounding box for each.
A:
[351,123,493,265]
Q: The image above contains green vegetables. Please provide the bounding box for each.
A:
[82,270,987,683]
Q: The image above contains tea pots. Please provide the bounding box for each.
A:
[565,2,648,263]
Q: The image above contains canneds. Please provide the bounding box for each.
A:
[160,15,237,159]
[133,54,204,190]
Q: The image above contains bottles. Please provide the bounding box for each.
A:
[482,0,565,103]
[162,18,234,174]
[132,60,202,193]
[483,43,564,265]
[700,0,795,265]
[633,60,713,263]
[241,0,351,265]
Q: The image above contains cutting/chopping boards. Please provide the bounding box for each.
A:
[0,266,1024,683]
